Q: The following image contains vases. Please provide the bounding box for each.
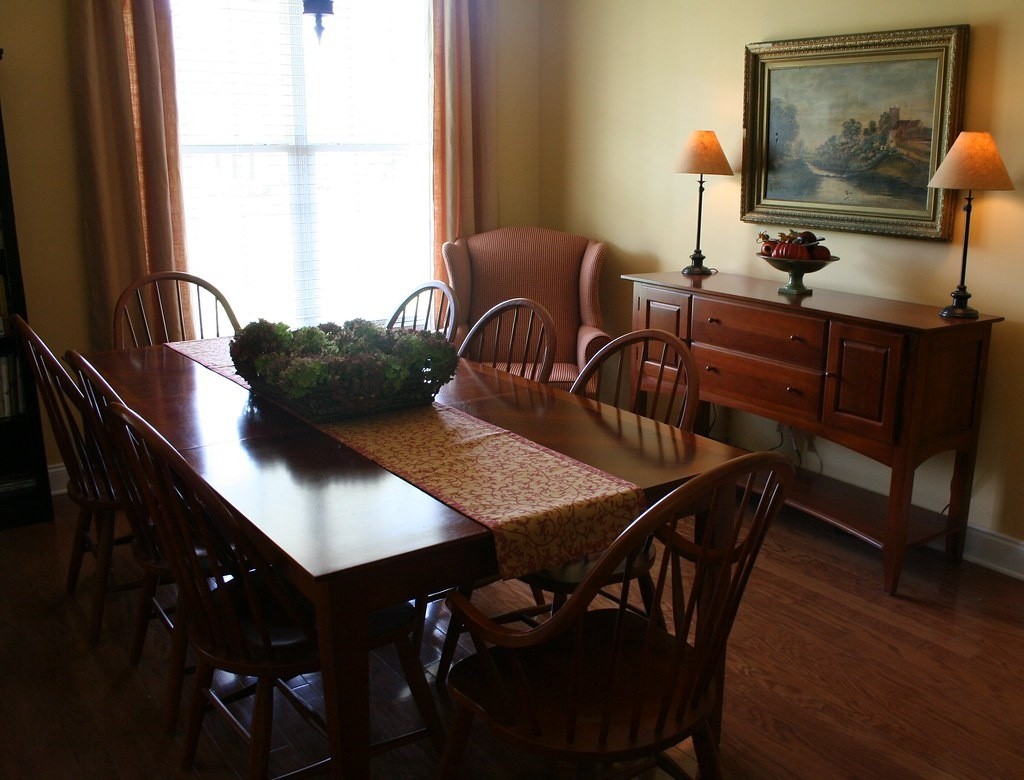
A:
[263,372,426,422]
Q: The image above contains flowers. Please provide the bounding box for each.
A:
[227,316,460,404]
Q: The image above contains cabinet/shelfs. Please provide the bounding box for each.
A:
[620,268,1006,596]
[0,46,56,533]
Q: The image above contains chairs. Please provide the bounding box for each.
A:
[441,228,611,400]
[12,271,795,780]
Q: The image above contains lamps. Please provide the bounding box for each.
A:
[933,144,1015,320]
[670,131,735,275]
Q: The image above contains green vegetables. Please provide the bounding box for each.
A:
[229,317,460,397]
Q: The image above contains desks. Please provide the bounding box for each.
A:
[58,337,752,780]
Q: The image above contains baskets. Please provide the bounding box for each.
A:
[250,372,449,421]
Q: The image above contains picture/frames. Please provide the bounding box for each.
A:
[741,24,970,242]
